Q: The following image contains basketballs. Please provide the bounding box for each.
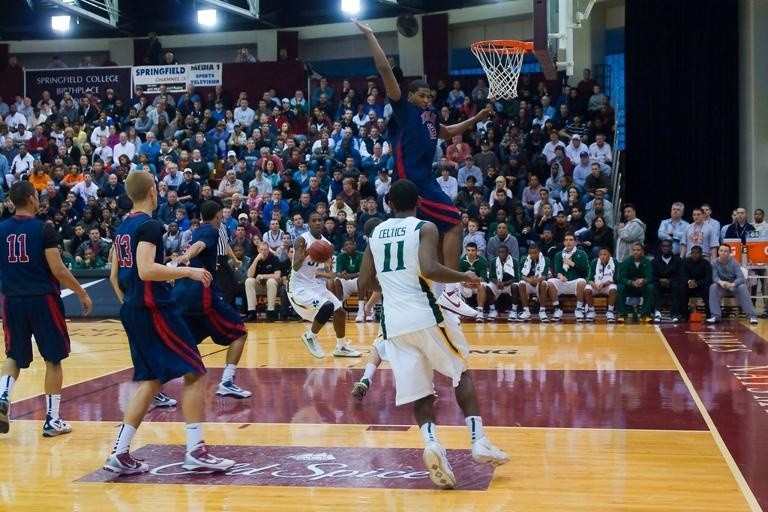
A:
[311,241,331,262]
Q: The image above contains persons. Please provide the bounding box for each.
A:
[352,217,383,400]
[149,201,252,408]
[0,179,92,436]
[358,179,508,489]
[287,211,363,358]
[102,171,235,474]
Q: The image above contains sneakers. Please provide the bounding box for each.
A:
[332,344,362,357]
[476,307,563,322]
[706,316,721,323]
[0,399,9,433]
[300,331,325,359]
[355,311,375,322]
[749,318,758,324]
[152,392,178,407]
[471,437,509,467]
[181,440,236,472]
[240,314,256,321]
[606,310,688,323]
[351,377,372,400]
[215,380,252,399]
[435,289,478,318]
[575,307,596,321]
[422,442,456,488]
[104,451,150,475]
[42,415,72,437]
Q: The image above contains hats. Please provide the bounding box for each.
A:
[184,168,193,174]
[238,213,249,220]
[228,150,236,158]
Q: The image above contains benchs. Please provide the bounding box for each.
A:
[249,289,741,323]
